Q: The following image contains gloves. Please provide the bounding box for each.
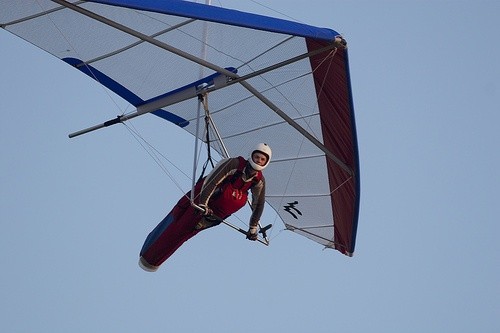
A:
[197,207,213,217]
[246,226,258,241]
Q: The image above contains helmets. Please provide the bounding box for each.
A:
[248,143,272,171]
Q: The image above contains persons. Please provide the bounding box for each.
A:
[139,143,272,272]
